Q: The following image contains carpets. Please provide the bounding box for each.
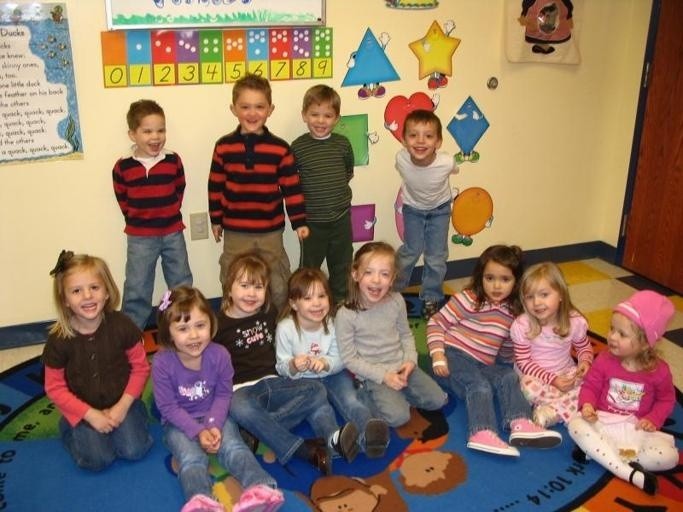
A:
[0,292,683,512]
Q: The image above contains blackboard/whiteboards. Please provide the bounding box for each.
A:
[106,0,327,30]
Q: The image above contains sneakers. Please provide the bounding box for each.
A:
[422,306,439,320]
[180,494,224,512]
[464,400,562,458]
[232,482,285,511]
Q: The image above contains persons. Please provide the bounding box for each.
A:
[208,74,311,326]
[567,289,680,497]
[391,109,459,320]
[290,82,355,315]
[110,97,194,334]
[152,285,285,512]
[509,260,596,430]
[40,248,155,471]
[332,241,449,429]
[273,266,391,464]
[425,244,563,458]
[211,252,334,478]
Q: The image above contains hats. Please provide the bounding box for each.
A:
[612,289,675,351]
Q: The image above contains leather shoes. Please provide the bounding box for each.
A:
[303,415,389,477]
[571,443,586,464]
[630,461,657,494]
[236,421,258,454]
